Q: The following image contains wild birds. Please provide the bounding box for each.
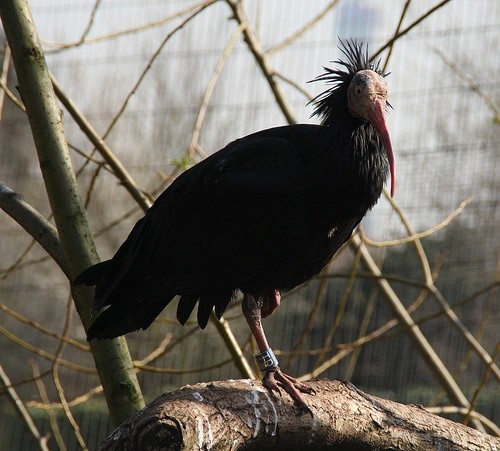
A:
[72,37,395,420]
